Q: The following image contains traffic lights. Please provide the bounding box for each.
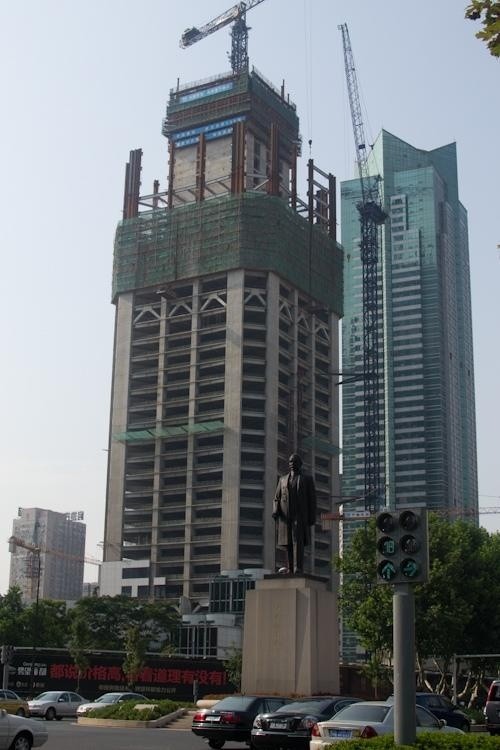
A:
[373,509,431,586]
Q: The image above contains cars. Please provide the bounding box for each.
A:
[26,690,92,722]
[245,694,363,750]
[189,691,293,750]
[0,707,50,750]
[0,688,31,718]
[387,691,471,733]
[307,699,470,750]
[75,691,151,720]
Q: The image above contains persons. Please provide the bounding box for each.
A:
[271,452,316,575]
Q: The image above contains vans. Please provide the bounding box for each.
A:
[483,677,500,737]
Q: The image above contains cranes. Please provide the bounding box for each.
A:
[320,503,500,523]
[338,21,398,513]
[175,0,267,74]
[3,534,102,601]
